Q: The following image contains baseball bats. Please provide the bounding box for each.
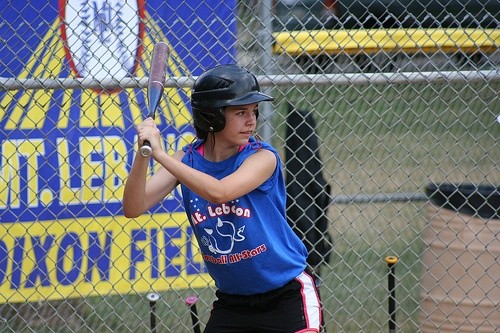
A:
[186,296,200,333]
[385,255,398,333]
[146,293,160,333]
[140,43,170,157]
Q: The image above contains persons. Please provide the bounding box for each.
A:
[121,61,325,333]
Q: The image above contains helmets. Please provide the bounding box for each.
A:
[190,65,275,141]
